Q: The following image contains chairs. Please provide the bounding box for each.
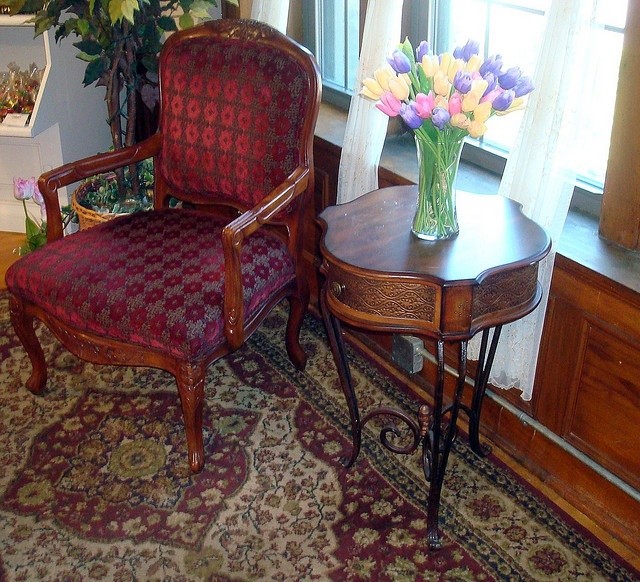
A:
[4,17,324,473]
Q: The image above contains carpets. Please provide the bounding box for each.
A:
[0,289,639,581]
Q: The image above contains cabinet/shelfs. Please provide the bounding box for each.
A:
[0,0,129,236]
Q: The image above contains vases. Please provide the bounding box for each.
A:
[410,134,465,242]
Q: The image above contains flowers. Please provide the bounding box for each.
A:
[12,176,47,256]
[358,37,535,238]
[12,178,82,257]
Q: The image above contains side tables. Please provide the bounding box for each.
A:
[315,184,552,554]
